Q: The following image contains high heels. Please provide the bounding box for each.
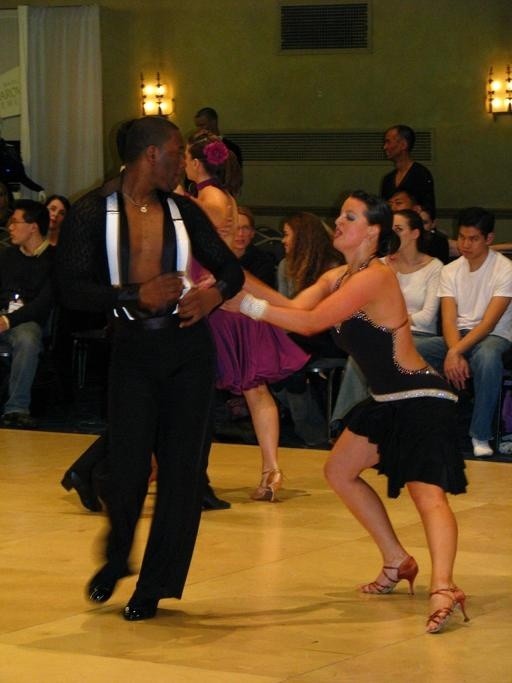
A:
[359,556,418,596]
[425,584,470,633]
[251,467,282,501]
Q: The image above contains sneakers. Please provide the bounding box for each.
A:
[499,442,512,454]
[472,437,493,457]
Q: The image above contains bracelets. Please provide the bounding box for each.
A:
[239,294,269,321]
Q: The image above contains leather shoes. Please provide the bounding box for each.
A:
[122,592,159,620]
[202,485,231,509]
[61,467,102,512]
[89,563,118,602]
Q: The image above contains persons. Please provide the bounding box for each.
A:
[387,190,422,216]
[0,199,57,430]
[419,204,436,230]
[331,210,442,431]
[60,433,230,512]
[183,132,309,504]
[233,207,270,286]
[66,117,245,622]
[41,195,70,245]
[417,206,512,459]
[378,125,437,206]
[272,212,338,300]
[193,108,243,190]
[220,191,470,635]
[0,136,47,198]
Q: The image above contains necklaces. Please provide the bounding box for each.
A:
[120,188,161,216]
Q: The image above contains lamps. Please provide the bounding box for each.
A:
[137,73,173,117]
[485,62,511,115]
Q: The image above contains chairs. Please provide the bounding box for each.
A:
[0,305,58,358]
[300,358,346,443]
[449,253,512,452]
[68,329,109,389]
